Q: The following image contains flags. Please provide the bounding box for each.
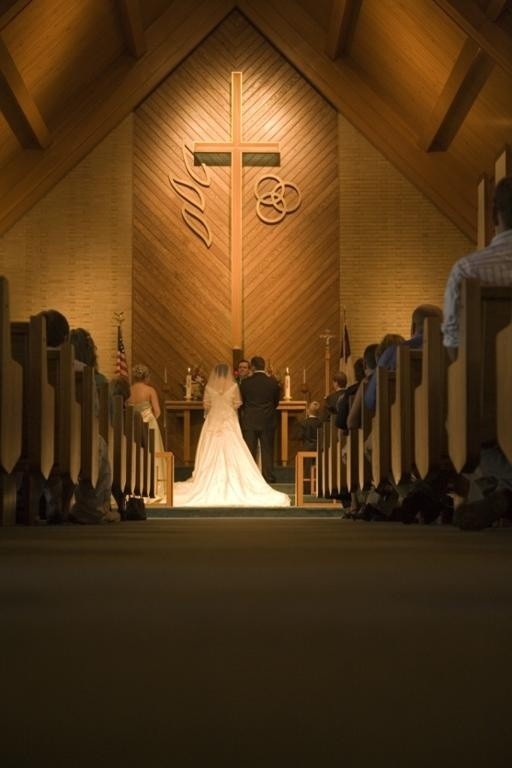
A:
[115,325,128,379]
[340,315,351,360]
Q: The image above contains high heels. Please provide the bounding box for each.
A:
[344,511,357,521]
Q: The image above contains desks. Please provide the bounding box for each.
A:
[164,399,307,467]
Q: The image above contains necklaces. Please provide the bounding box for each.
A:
[238,356,279,484]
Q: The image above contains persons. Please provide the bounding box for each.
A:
[161,364,291,507]
[323,302,446,523]
[108,372,131,409]
[440,174,512,530]
[234,359,249,386]
[70,326,128,521]
[126,363,167,502]
[32,309,122,525]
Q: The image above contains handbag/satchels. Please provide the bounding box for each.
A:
[126,498,147,520]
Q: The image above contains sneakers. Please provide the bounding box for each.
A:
[357,507,367,518]
[104,512,121,522]
[453,488,512,530]
